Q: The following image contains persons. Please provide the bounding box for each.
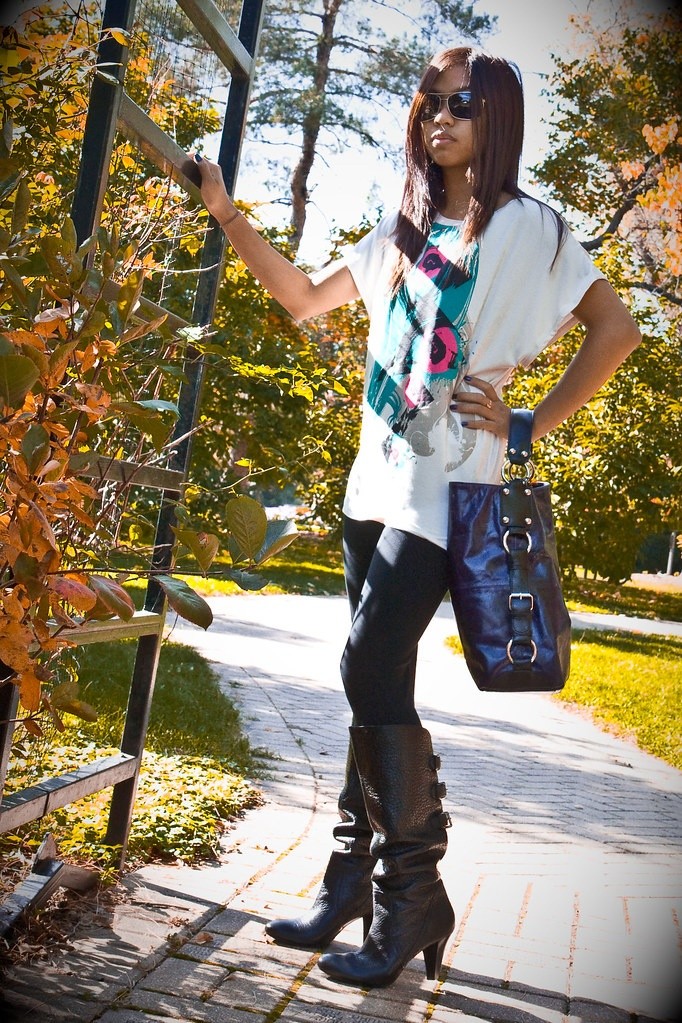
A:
[187,45,643,984]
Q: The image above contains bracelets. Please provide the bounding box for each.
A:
[221,210,240,229]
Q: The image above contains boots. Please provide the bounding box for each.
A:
[316,724,455,987]
[265,737,373,949]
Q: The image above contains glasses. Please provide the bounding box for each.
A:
[420,91,485,122]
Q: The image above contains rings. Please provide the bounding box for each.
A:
[486,400,493,409]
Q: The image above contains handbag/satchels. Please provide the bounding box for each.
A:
[449,409,570,692]
